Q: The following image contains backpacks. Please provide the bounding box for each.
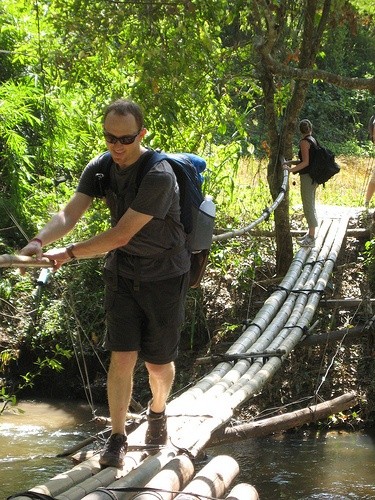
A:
[304,138,340,184]
[97,151,216,288]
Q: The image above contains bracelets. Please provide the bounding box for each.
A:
[30,238,42,246]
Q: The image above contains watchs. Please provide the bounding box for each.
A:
[66,244,75,258]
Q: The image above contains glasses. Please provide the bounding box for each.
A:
[103,128,143,145]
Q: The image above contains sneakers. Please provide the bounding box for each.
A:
[296,235,316,247]
[144,399,167,455]
[99,432,128,467]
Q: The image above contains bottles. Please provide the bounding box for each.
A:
[194,195,217,248]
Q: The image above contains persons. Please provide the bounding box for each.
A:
[280,118,319,247]
[365,114,375,208]
[20,99,191,467]
